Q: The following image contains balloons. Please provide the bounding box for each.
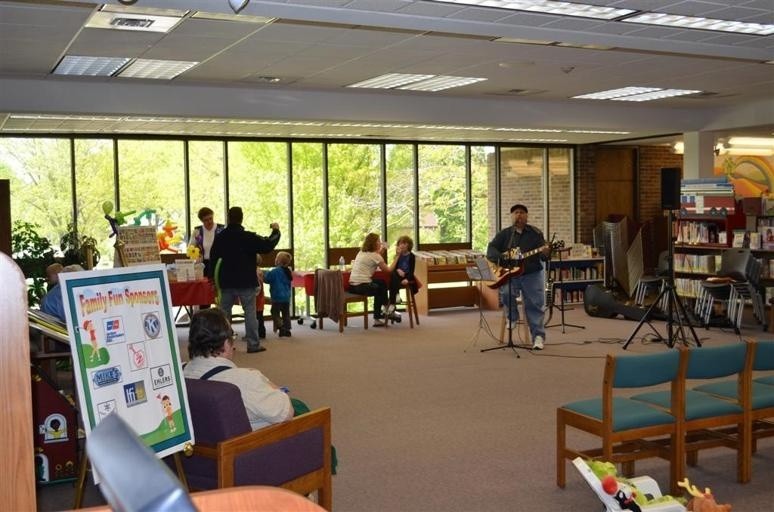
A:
[102,201,200,261]
[213,257,222,306]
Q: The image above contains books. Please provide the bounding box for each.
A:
[547,242,604,303]
[415,248,484,266]
[671,197,773,309]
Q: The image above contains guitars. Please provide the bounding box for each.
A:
[480,240,565,288]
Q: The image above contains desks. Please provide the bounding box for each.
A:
[290,270,421,329]
[170,278,265,328]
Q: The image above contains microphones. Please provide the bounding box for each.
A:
[513,220,519,227]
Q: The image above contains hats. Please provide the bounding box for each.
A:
[510,204,528,214]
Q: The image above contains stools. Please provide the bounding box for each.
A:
[385,279,423,330]
[499,285,532,344]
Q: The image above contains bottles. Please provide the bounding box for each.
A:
[339,256,345,271]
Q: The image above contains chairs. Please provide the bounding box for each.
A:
[633,251,672,311]
[556,346,685,499]
[632,339,752,487]
[316,269,369,333]
[181,378,334,511]
[695,337,774,481]
[696,248,767,332]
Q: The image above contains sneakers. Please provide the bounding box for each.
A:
[533,335,544,349]
[507,320,517,330]
[247,346,266,352]
[387,305,396,316]
[373,319,384,326]
[279,331,291,337]
[273,318,283,331]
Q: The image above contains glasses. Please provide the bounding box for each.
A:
[232,333,239,339]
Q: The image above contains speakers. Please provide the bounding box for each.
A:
[661,167,681,210]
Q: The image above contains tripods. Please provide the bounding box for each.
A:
[480,254,532,358]
[463,281,506,354]
[622,210,702,349]
[545,250,585,333]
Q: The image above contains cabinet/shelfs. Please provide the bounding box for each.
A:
[412,251,500,317]
[548,257,606,309]
[669,196,774,324]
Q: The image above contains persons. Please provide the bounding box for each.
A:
[44,263,64,293]
[189,207,226,310]
[486,205,546,350]
[383,235,415,317]
[347,234,402,327]
[207,206,281,354]
[262,251,293,337]
[241,253,265,341]
[180,307,337,501]
[39,263,85,323]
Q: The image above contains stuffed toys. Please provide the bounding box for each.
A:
[586,459,732,512]
